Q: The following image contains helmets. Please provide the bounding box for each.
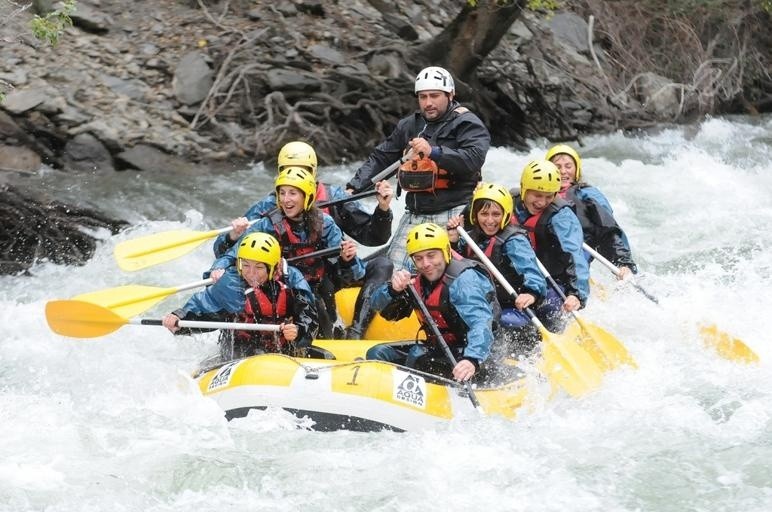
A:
[519,160,562,202]
[236,231,281,281]
[414,67,455,99]
[274,166,319,212]
[546,144,582,182]
[405,222,452,265]
[469,183,514,230]
[277,141,319,181]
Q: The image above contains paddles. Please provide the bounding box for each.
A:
[534,257,639,375]
[68,244,344,318]
[44,300,288,339]
[582,242,761,365]
[113,189,388,273]
[448,223,602,399]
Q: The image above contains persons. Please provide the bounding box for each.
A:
[446,184,548,363]
[161,232,336,361]
[507,159,591,334]
[210,167,366,340]
[212,142,394,260]
[546,144,638,282]
[365,223,502,390]
[344,67,490,274]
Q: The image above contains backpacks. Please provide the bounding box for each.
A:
[392,106,483,202]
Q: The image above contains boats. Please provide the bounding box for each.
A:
[190,275,638,433]
